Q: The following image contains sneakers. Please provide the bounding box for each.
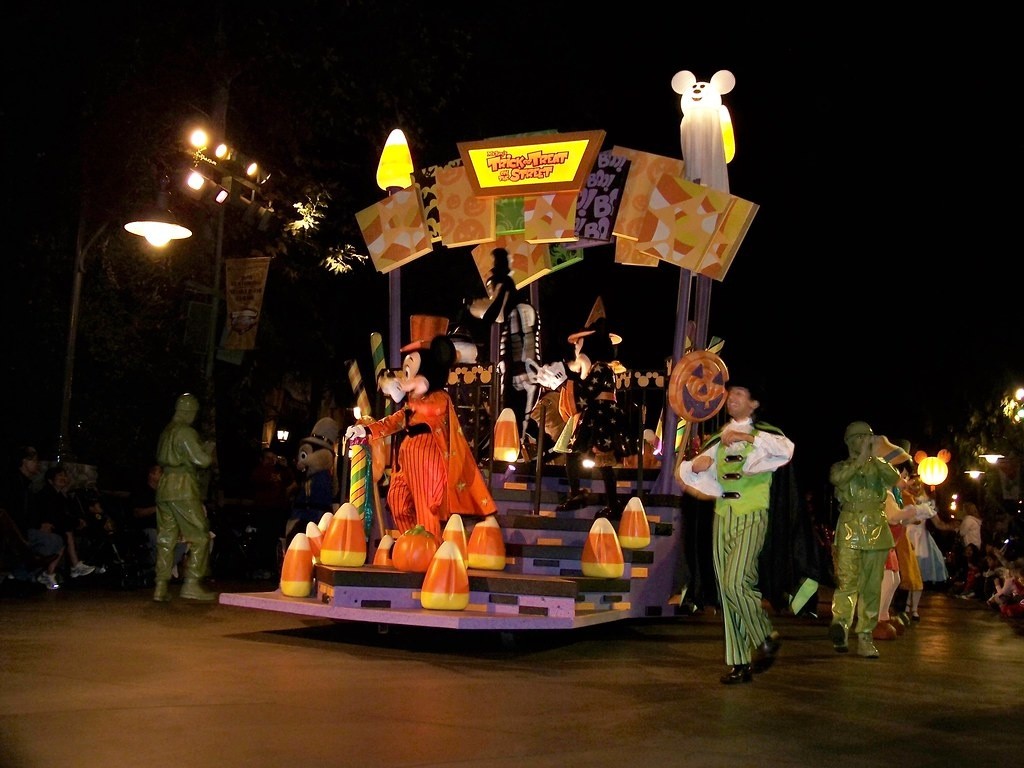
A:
[180,583,217,601]
[37,572,59,590]
[155,586,172,602]
[856,633,880,658]
[831,623,849,653]
[71,561,95,578]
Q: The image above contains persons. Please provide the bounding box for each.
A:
[829,422,902,656]
[591,421,722,623]
[671,70,736,194]
[524,381,568,467]
[154,393,216,602]
[804,435,1024,640]
[0,444,355,590]
[679,372,794,686]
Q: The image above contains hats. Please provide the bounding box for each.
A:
[724,346,770,400]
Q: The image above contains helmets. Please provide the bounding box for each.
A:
[844,421,873,437]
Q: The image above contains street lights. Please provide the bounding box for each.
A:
[180,131,269,436]
[62,163,194,449]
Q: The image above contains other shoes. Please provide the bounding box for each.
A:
[959,589,975,599]
[750,637,782,674]
[720,663,753,684]
[911,610,920,621]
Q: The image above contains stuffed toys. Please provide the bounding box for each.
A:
[285,418,338,547]
[464,248,561,465]
[537,297,625,521]
[346,335,498,559]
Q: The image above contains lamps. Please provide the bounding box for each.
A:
[914,448,952,493]
